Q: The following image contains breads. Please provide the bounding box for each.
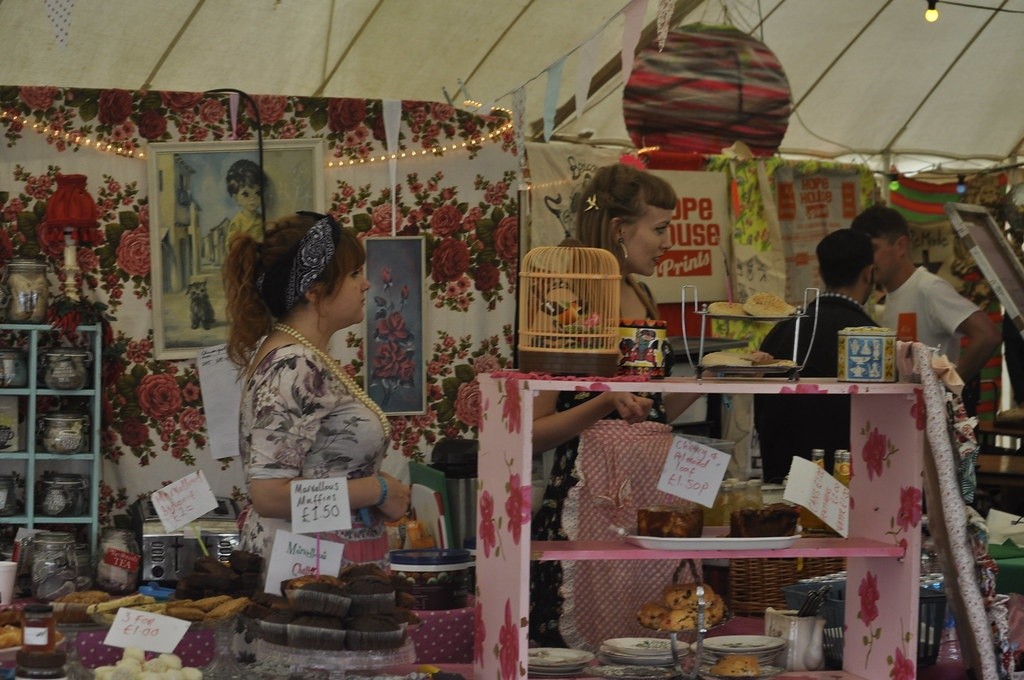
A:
[708,654,760,677]
[707,292,797,316]
[636,580,724,632]
[0,588,252,650]
[701,349,774,367]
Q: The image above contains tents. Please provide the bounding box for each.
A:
[0,1,1024,618]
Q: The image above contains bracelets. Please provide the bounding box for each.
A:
[374,475,388,507]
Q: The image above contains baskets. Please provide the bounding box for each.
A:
[727,528,847,614]
[781,580,947,667]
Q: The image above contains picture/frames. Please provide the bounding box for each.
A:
[363,236,427,417]
[146,138,324,360]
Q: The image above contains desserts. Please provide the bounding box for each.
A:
[238,562,421,650]
[729,502,797,538]
[636,505,703,538]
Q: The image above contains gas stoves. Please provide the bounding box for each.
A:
[600,636,690,667]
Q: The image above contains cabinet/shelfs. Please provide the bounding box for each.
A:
[0,318,103,591]
[475,373,926,680]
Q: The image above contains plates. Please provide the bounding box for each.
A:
[526,646,596,675]
[691,634,788,677]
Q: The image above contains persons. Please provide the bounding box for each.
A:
[526,162,774,652]
[851,205,1005,385]
[219,210,419,581]
[749,226,882,485]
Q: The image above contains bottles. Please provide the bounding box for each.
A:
[800,449,825,533]
[702,478,763,525]
[935,601,963,667]
[830,449,851,533]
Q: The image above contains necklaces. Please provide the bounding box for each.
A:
[274,322,392,439]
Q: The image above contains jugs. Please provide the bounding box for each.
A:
[764,606,828,670]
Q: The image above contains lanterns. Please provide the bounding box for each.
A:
[618,21,794,175]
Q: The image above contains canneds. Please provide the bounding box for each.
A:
[20,605,55,656]
